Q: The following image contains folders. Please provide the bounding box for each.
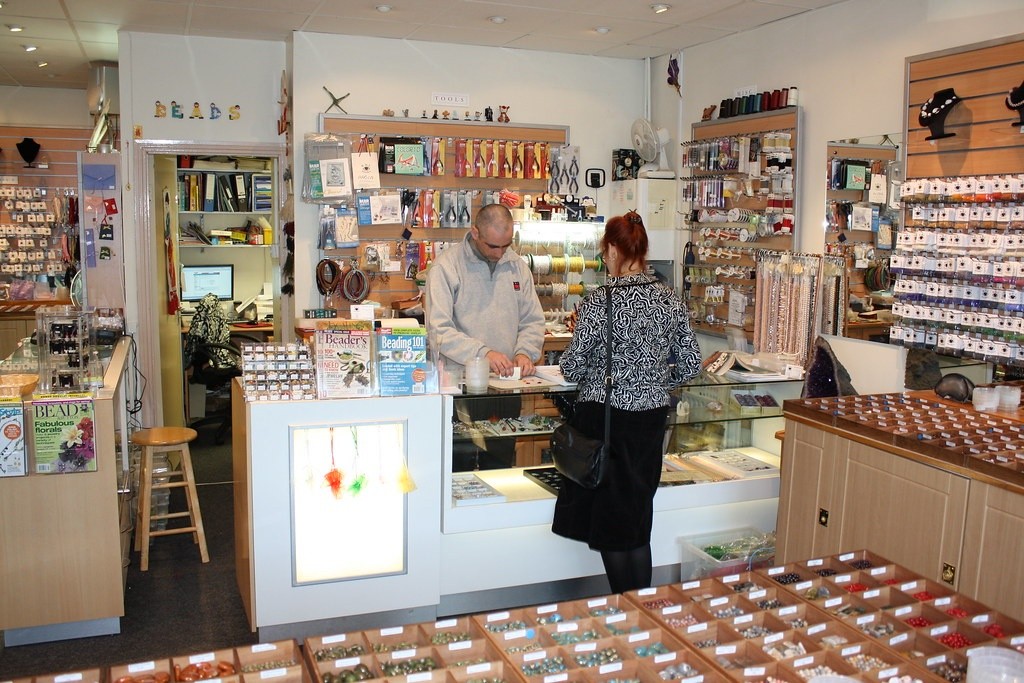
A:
[204,174,215,213]
[235,174,247,212]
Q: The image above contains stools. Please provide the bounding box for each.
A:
[131,427,210,571]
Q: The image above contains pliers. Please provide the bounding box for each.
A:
[433,150,579,193]
[413,199,420,219]
[446,199,456,222]
[459,200,470,223]
[428,199,439,223]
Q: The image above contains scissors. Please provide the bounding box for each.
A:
[403,190,415,227]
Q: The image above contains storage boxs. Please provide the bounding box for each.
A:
[678,527,776,584]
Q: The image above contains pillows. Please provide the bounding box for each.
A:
[188,292,236,370]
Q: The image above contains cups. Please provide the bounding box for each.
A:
[439,365,463,395]
[465,357,490,393]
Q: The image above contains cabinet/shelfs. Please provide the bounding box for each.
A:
[0,335,135,647]
[228,363,812,642]
[176,168,274,248]
[779,391,1024,628]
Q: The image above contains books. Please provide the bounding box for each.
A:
[375,327,427,397]
[702,350,754,376]
[315,319,379,399]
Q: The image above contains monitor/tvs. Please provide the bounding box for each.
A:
[180,264,234,302]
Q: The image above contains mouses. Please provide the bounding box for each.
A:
[247,320,259,325]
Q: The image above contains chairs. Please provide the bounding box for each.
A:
[182,332,261,444]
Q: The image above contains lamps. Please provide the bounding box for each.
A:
[653,6,668,13]
[24,45,36,52]
[37,61,48,67]
[9,24,23,32]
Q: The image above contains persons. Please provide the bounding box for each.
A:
[424,204,546,470]
[552,209,702,595]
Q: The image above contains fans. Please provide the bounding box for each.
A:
[631,118,676,178]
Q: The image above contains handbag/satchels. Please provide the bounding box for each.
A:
[550,423,607,487]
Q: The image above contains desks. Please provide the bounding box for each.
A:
[179,320,273,333]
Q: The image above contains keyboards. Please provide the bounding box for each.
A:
[225,317,253,324]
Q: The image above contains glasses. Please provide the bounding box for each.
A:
[600,249,608,264]
